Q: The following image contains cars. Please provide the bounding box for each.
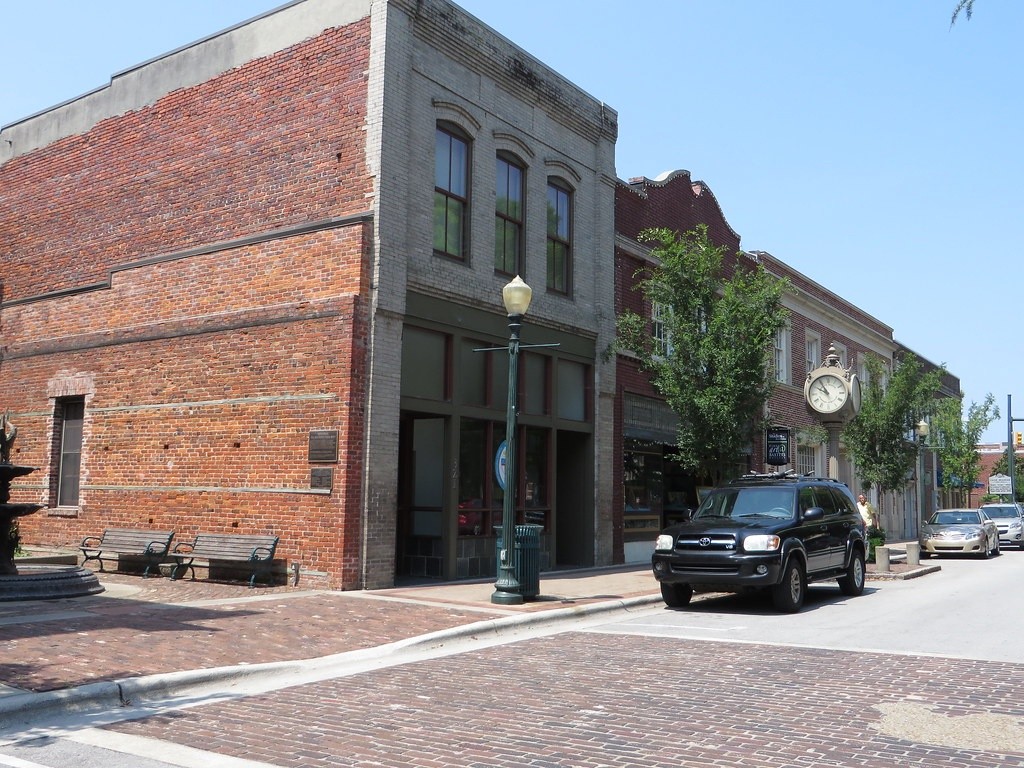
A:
[918,508,1000,560]
[458,497,506,538]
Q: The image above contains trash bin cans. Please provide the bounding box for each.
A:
[493,524,545,602]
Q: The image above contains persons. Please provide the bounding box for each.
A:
[857,495,879,529]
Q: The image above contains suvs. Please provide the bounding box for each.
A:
[652,469,868,615]
[978,501,1024,550]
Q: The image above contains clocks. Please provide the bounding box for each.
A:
[808,373,848,413]
[852,374,862,414]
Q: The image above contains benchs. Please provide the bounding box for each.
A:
[167,533,280,589]
[78,527,176,581]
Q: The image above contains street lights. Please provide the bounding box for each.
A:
[917,419,929,526]
[489,275,533,605]
